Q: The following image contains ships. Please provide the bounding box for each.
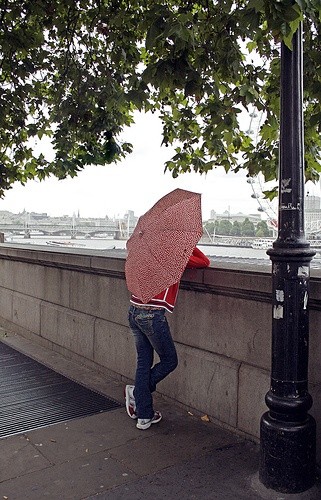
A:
[249,238,275,250]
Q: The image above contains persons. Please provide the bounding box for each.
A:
[125,244,210,431]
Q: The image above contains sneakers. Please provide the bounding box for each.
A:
[125,385,136,420]
[136,411,161,430]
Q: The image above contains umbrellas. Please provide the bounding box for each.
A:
[125,189,203,304]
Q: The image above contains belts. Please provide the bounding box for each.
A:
[132,304,163,311]
